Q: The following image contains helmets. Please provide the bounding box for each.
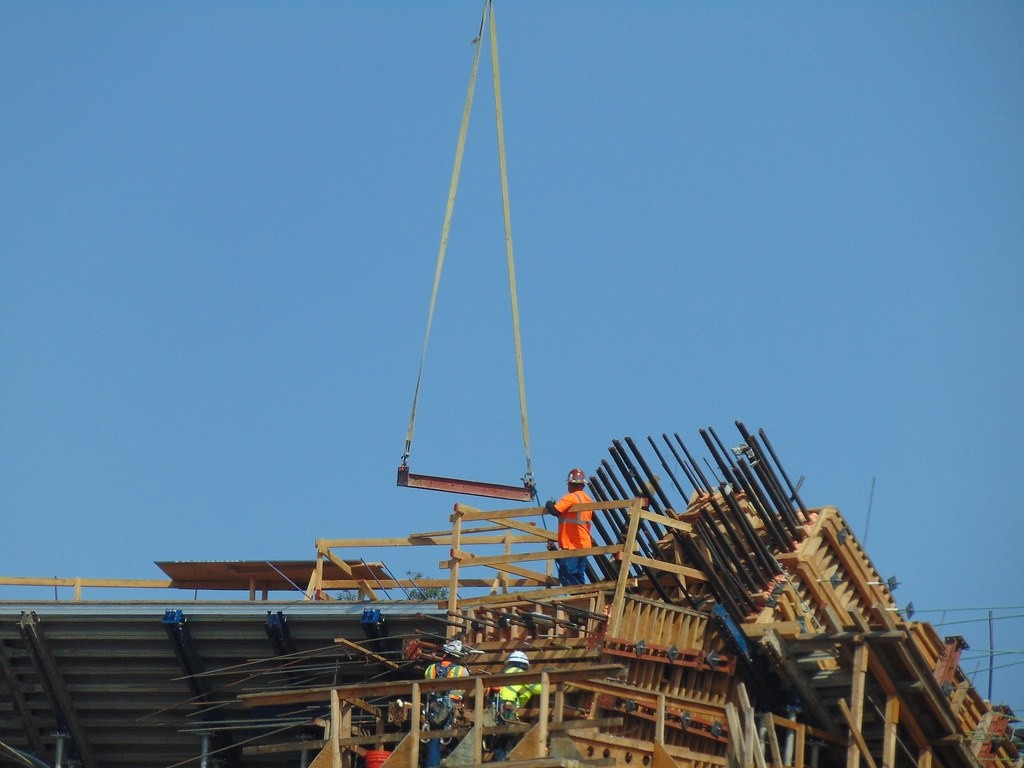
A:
[445,640,467,659]
[566,469,587,484]
[505,651,531,667]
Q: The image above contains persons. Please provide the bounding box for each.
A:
[483,650,541,763]
[423,639,468,768]
[546,467,593,587]
